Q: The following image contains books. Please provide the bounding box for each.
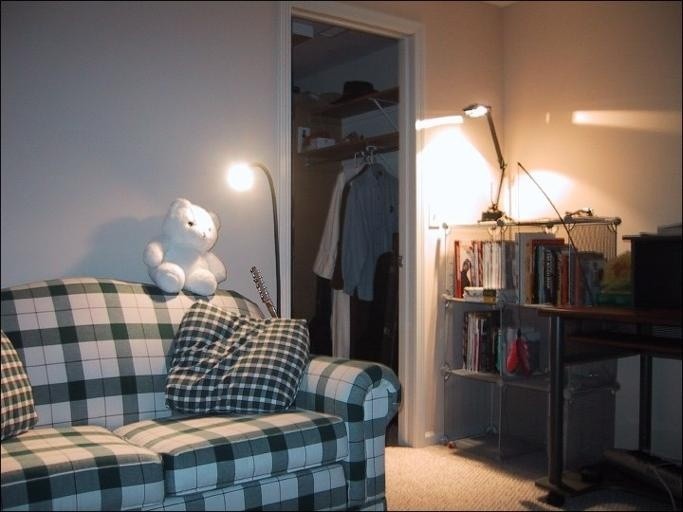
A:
[657,220,683,237]
[445,230,609,382]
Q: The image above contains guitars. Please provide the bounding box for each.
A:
[250,265,277,318]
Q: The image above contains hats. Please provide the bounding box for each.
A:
[330,79,378,102]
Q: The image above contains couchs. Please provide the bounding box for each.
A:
[1,276,404,512]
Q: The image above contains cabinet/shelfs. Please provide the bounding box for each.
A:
[306,85,399,165]
[439,217,624,466]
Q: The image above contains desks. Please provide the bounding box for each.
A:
[522,297,682,509]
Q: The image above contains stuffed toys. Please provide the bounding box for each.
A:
[142,196,228,297]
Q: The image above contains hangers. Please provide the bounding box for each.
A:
[335,143,398,190]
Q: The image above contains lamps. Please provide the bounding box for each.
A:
[462,102,510,224]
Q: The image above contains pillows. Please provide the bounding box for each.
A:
[1,332,40,440]
[165,297,309,416]
